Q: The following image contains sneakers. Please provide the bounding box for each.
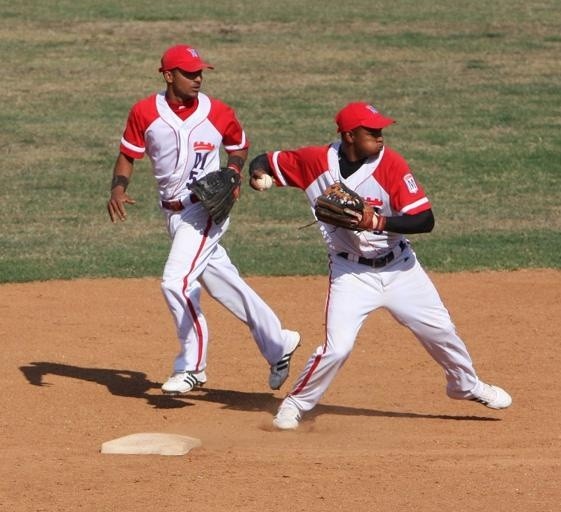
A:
[272,395,304,431]
[161,368,207,396]
[268,329,304,390]
[446,380,512,410]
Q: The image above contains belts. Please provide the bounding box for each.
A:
[162,192,201,212]
[336,240,408,268]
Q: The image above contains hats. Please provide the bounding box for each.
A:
[158,44,215,73]
[335,101,397,134]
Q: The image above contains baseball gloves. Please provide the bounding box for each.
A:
[315,181,375,233]
[189,166,241,225]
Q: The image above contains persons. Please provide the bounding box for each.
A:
[107,45,300,393]
[249,101,512,432]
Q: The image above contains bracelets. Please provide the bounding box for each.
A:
[111,175,128,192]
[228,155,245,171]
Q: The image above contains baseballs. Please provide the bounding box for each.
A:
[255,173,273,191]
